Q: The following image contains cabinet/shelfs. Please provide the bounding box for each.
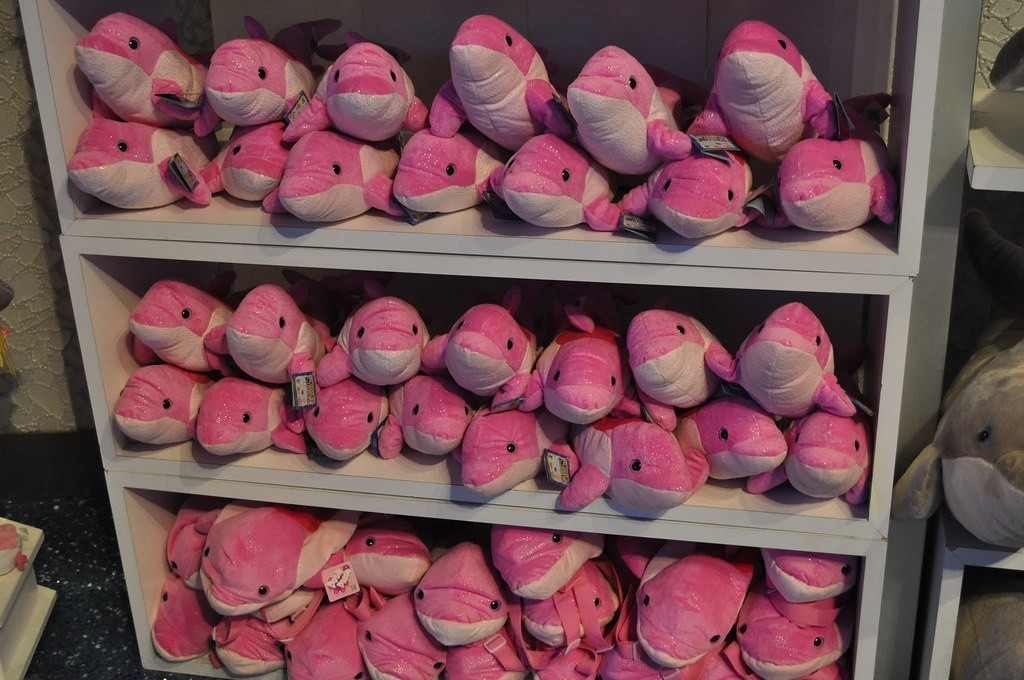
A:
[19,0,943,680]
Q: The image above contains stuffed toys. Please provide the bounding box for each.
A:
[947,587,1024,680]
[965,34,1024,155]
[0,523,30,578]
[63,12,901,680]
[888,208,1024,548]
